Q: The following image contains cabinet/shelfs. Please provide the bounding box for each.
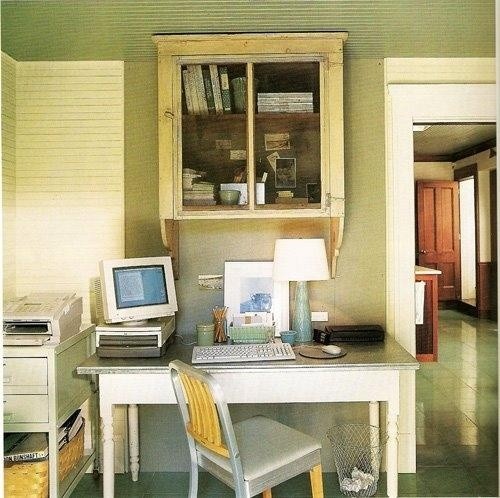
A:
[152,30,350,280]
[3,322,97,497]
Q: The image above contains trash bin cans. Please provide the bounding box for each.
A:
[327,423,389,498]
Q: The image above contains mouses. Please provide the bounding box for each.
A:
[322,345,341,355]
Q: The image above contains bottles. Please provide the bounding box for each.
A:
[196,323,215,347]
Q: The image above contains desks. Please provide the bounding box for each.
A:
[76,334,421,497]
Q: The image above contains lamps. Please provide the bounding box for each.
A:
[272,237,332,343]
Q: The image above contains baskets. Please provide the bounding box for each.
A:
[4,417,85,498]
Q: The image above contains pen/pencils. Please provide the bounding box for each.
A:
[212,306,229,343]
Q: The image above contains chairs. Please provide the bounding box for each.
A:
[169,359,327,497]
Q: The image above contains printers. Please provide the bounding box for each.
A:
[3,288,84,347]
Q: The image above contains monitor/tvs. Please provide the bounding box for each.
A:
[100,255,179,325]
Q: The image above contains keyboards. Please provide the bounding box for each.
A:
[191,342,295,365]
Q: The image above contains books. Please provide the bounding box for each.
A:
[177,62,320,212]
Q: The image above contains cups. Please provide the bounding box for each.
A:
[280,331,297,345]
[219,189,241,206]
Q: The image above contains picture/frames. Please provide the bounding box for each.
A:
[223,261,291,339]
